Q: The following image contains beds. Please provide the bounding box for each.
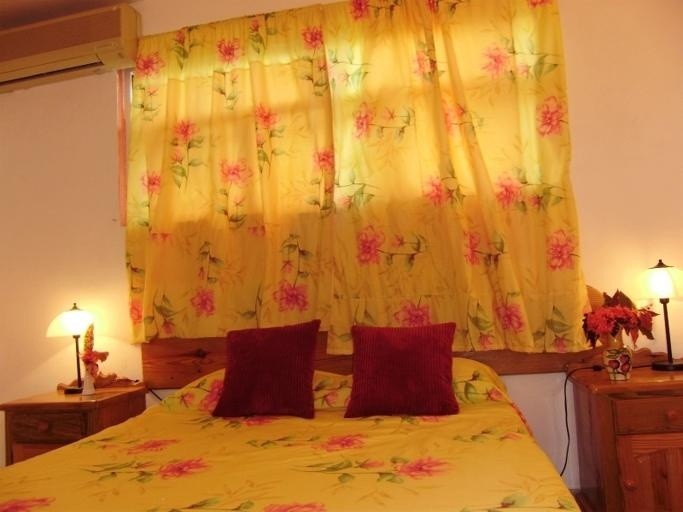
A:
[0,357,594,511]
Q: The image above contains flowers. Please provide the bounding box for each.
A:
[579,283,659,348]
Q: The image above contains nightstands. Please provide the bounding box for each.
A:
[0,384,148,466]
[556,363,682,511]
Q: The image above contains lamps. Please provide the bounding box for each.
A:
[47,300,97,395]
[629,258,683,371]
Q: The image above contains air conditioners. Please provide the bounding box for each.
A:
[0,3,141,96]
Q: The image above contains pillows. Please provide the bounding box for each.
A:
[344,321,459,417]
[210,319,319,420]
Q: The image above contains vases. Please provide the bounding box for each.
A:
[604,328,632,381]
